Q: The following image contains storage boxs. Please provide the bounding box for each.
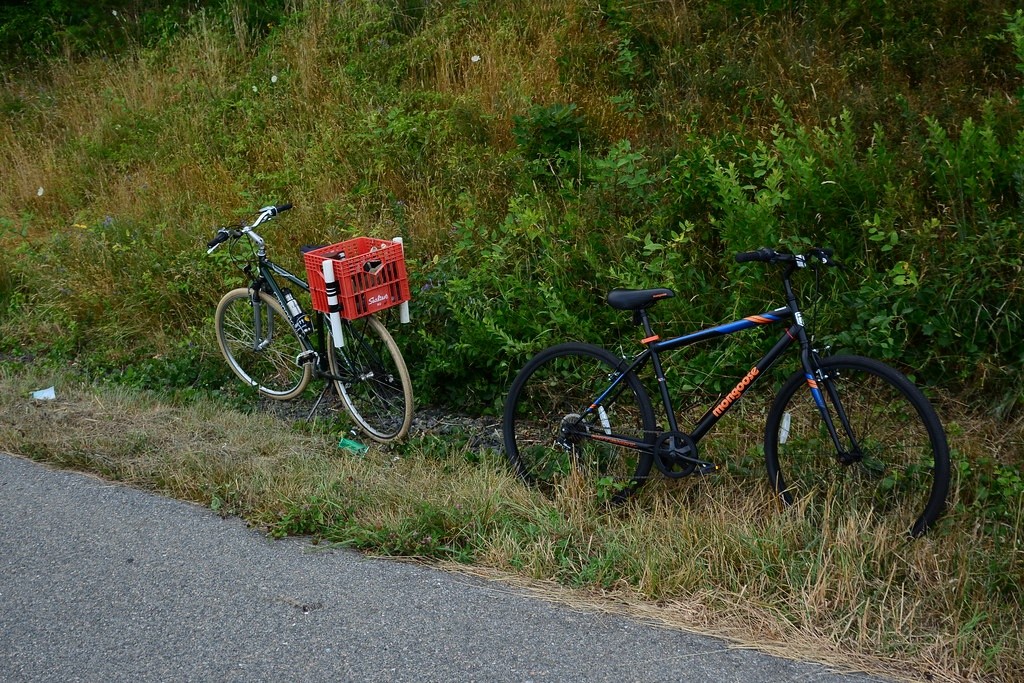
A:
[304,236,410,320]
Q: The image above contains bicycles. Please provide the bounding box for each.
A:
[499,246,954,544]
[206,203,414,449]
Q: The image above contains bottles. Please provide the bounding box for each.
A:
[281,287,312,334]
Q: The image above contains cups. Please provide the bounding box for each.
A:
[32,386,57,401]
[339,438,370,458]
